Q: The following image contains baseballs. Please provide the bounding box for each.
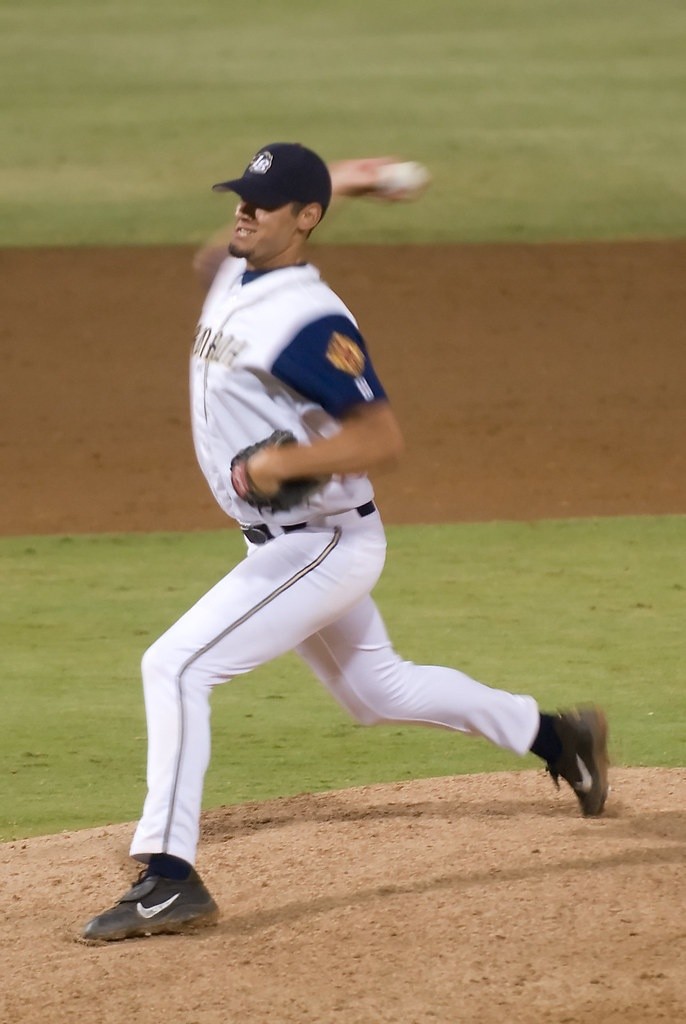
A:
[382,160,428,193]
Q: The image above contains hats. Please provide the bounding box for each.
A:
[212,142,333,211]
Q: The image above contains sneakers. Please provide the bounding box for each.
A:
[83,868,219,940]
[546,701,609,817]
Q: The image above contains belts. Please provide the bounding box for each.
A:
[240,501,377,543]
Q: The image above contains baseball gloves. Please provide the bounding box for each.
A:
[229,430,319,510]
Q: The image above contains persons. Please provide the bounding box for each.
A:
[83,142,609,941]
